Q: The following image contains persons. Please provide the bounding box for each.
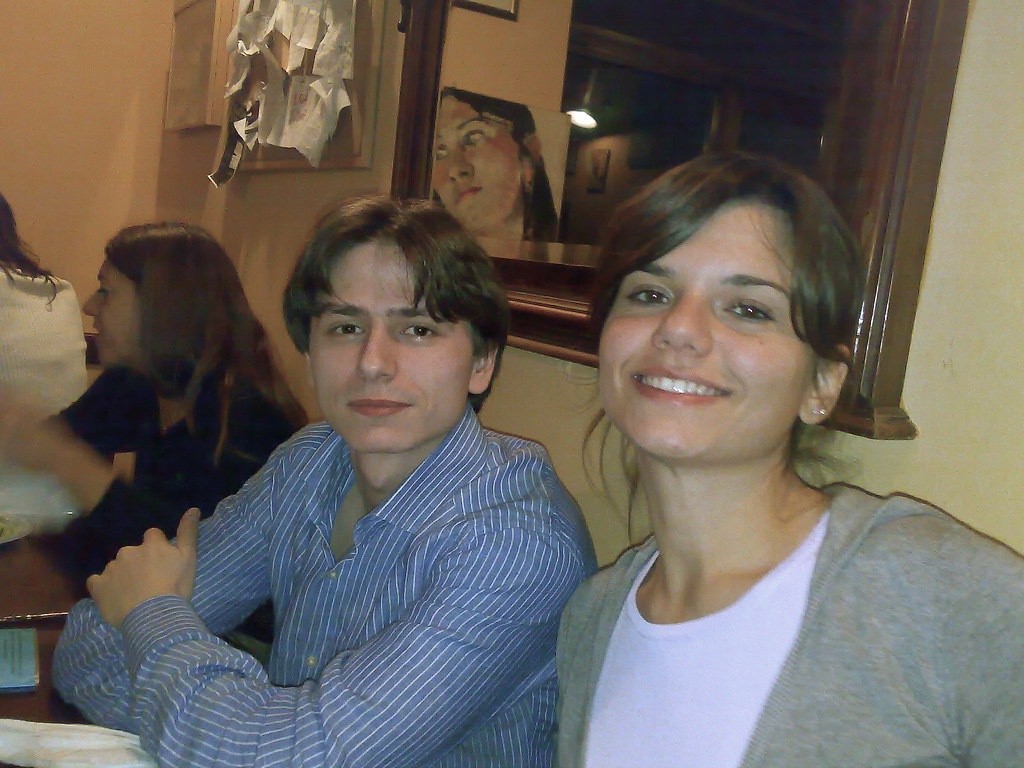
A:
[0,221,310,598]
[0,179,117,524]
[51,195,597,768]
[556,150,1024,768]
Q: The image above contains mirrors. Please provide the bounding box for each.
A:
[389,0,971,441]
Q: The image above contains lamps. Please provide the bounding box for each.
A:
[562,61,608,131]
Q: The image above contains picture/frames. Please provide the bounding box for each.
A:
[451,0,520,22]
